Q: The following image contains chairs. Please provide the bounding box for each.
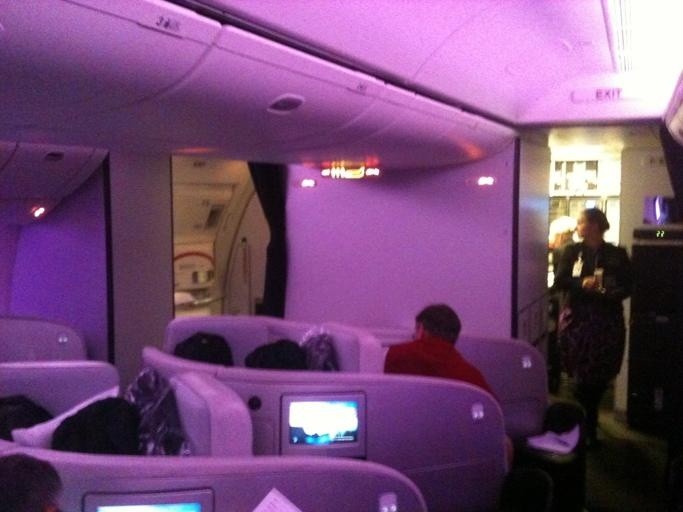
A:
[0,315,547,512]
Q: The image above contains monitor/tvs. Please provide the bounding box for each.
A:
[280,391,368,461]
[82,487,214,512]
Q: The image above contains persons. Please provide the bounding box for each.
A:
[50,394,142,457]
[551,206,636,442]
[243,338,306,371]
[0,451,64,512]
[0,393,54,442]
[173,330,236,369]
[383,303,501,407]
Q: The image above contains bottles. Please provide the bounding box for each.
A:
[594,268,603,293]
